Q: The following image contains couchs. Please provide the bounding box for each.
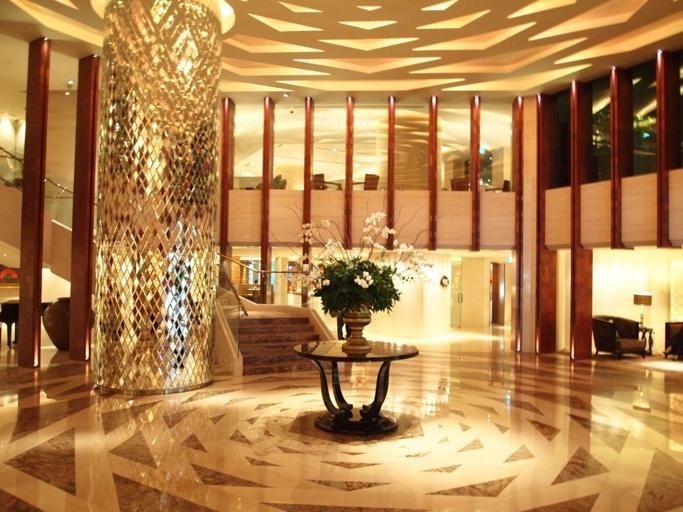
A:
[664,320,683,360]
[588,308,651,364]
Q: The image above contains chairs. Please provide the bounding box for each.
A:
[246,169,513,195]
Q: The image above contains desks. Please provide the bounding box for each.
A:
[287,336,422,437]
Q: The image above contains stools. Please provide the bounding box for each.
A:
[1,299,52,347]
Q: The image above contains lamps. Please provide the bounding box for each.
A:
[632,284,655,356]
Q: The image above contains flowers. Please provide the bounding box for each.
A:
[280,201,435,314]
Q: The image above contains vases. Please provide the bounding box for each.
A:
[40,293,98,354]
[336,308,380,360]
